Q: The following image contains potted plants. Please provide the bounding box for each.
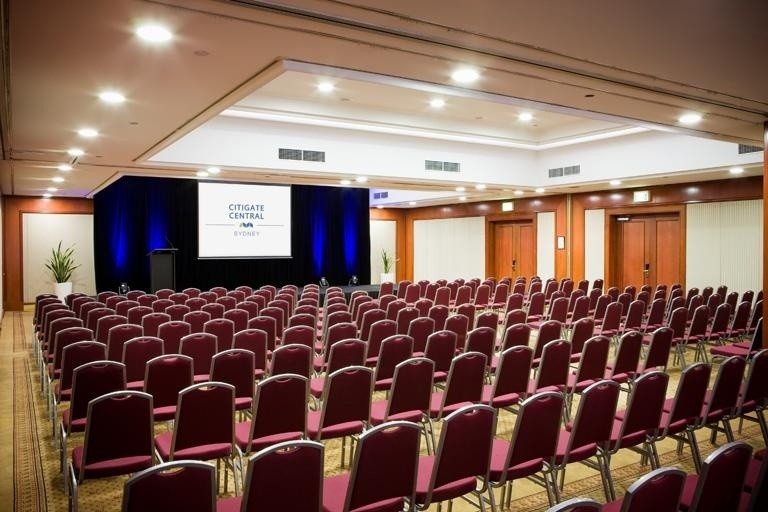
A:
[379,249,397,283]
[47,241,82,301]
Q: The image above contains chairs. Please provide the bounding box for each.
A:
[29,278,767,510]
